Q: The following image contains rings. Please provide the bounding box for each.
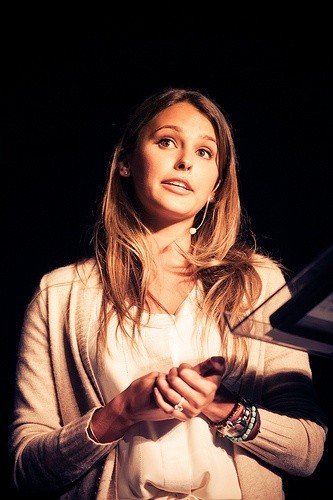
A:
[175,397,185,412]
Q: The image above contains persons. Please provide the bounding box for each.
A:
[12,90,330,497]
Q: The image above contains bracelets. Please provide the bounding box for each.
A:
[208,404,257,443]
